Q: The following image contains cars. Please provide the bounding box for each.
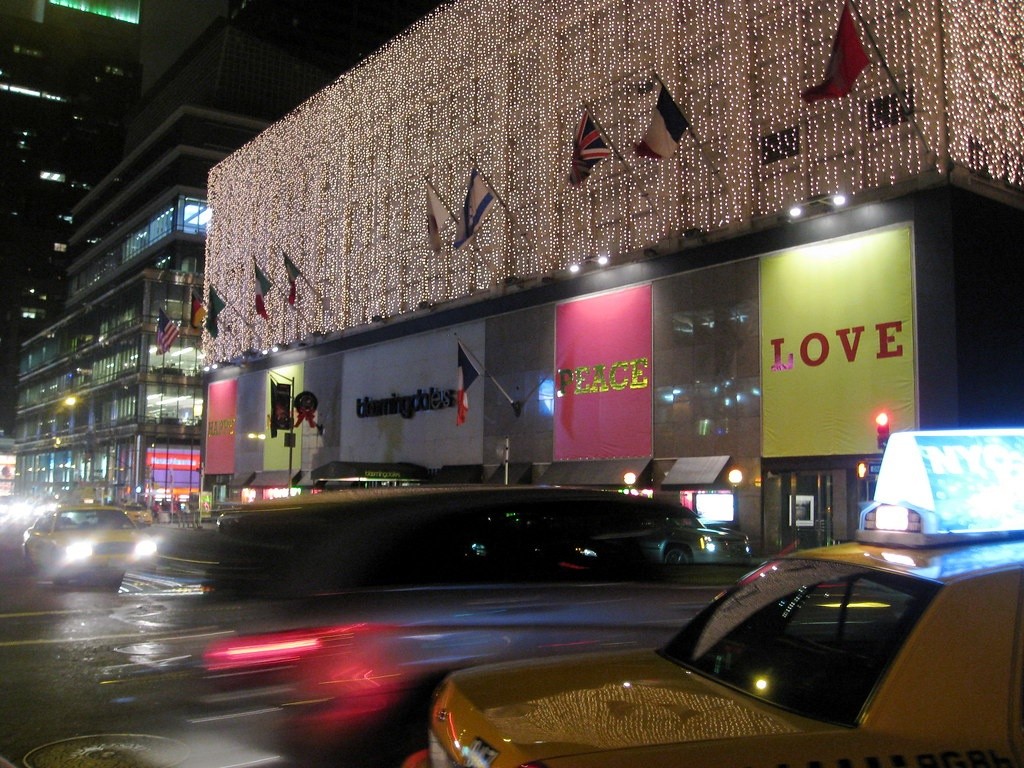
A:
[397,428,1024,767]
[22,504,157,590]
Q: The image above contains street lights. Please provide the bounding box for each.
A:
[729,469,743,531]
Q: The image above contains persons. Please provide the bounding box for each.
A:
[124,491,203,529]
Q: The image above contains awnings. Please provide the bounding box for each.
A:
[250,470,298,488]
[661,456,732,488]
[437,465,483,485]
[296,470,323,488]
[228,470,255,489]
[541,457,652,486]
[490,461,533,486]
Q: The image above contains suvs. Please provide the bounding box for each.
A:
[115,485,755,768]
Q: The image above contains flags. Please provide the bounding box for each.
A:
[270,378,277,438]
[206,284,228,339]
[802,0,869,104]
[568,106,614,189]
[634,73,689,160]
[155,305,179,355]
[425,181,450,256]
[454,164,496,252]
[189,292,206,332]
[456,340,483,427]
[254,262,274,321]
[282,251,301,306]
